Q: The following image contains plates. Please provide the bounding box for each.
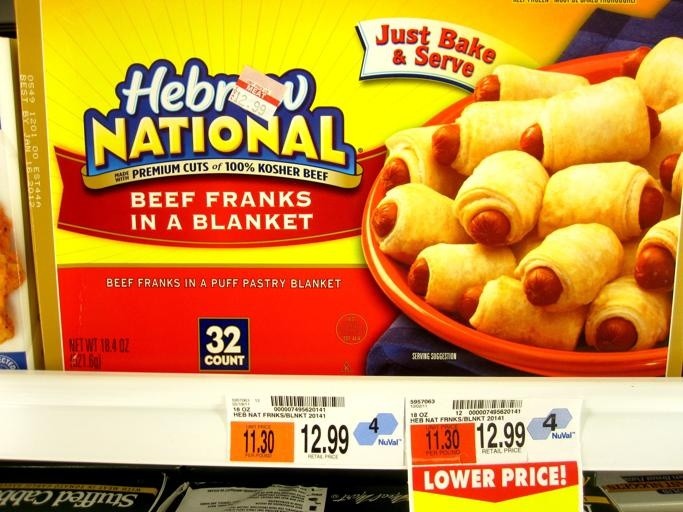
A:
[360,48,670,377]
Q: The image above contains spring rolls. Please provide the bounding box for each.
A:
[372,47,683,353]
